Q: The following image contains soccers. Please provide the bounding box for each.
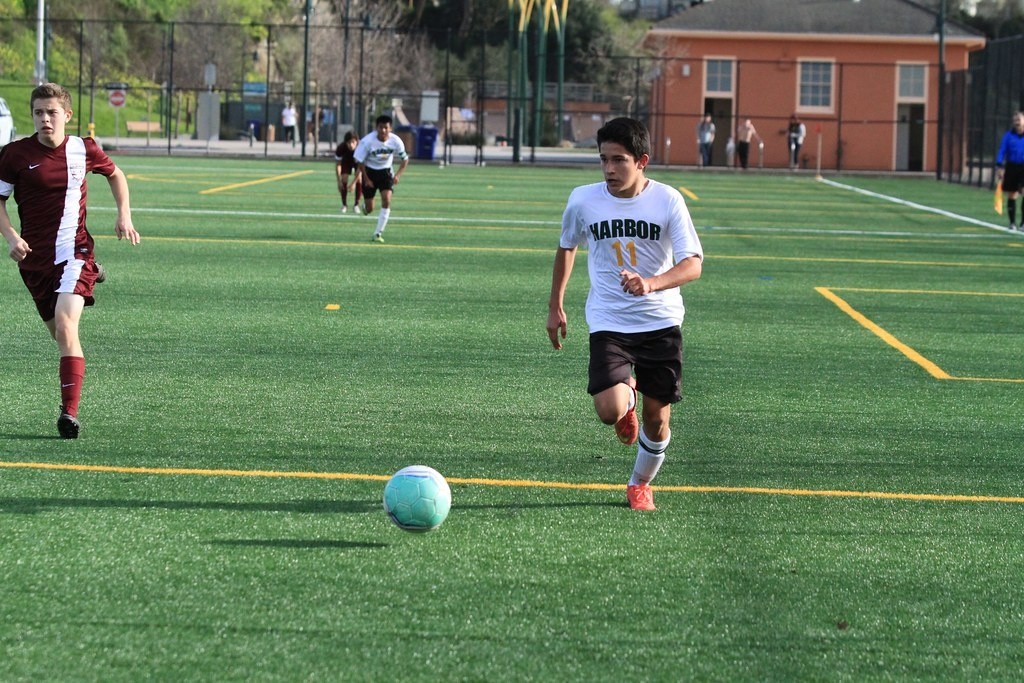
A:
[383,465,451,533]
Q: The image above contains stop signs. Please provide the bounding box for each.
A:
[109,90,125,108]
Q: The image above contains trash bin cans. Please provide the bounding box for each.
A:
[417,125,439,159]
[396,125,417,158]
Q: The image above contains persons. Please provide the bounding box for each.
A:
[737,117,762,168]
[787,116,806,169]
[347,116,408,243]
[335,130,364,213]
[0,81,140,439]
[311,105,324,141]
[548,117,703,511]
[995,110,1024,230]
[282,101,298,144]
[697,113,715,166]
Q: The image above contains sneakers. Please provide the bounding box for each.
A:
[362,209,367,216]
[372,233,385,242]
[615,377,639,445]
[626,482,656,510]
[57,405,80,439]
[95,261,106,283]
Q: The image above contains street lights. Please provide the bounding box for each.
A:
[300,1,315,155]
[936,0,977,179]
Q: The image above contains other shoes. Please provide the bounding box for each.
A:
[340,205,348,213]
[1008,223,1017,230]
[353,206,361,214]
[1019,224,1024,231]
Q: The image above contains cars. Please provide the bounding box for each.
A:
[0,97,14,147]
